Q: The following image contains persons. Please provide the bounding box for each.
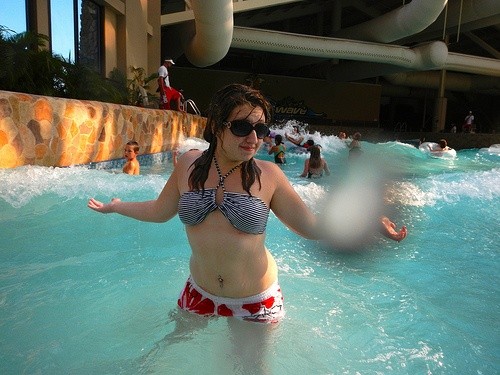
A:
[300,145,329,179]
[465,109,475,134]
[172,147,178,165]
[85,84,409,325]
[449,122,457,134]
[281,123,361,158]
[123,140,140,175]
[427,139,450,152]
[420,128,427,145]
[158,56,185,112]
[268,134,287,164]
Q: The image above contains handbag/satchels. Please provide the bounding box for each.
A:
[156,86,163,93]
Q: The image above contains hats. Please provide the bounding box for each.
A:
[163,59,175,65]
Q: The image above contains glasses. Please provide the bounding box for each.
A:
[223,118,271,140]
